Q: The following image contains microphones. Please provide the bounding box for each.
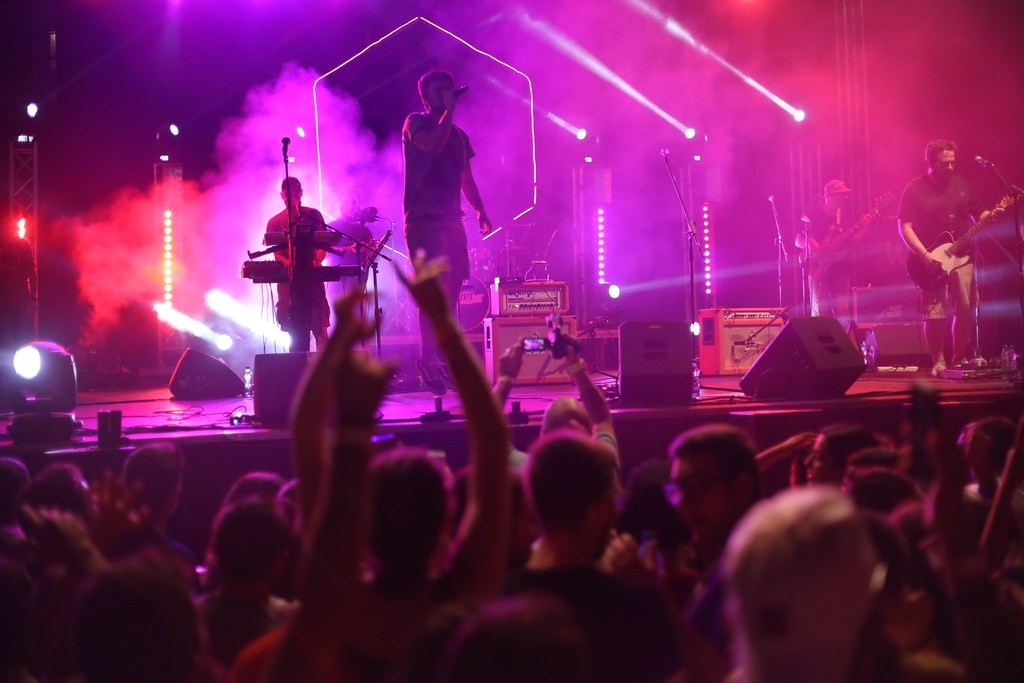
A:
[975,156,994,167]
[801,215,810,223]
[282,137,290,153]
[734,340,755,345]
[769,196,775,207]
[454,85,470,97]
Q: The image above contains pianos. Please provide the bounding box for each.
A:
[241,231,363,352]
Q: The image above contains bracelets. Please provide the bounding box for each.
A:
[919,251,928,259]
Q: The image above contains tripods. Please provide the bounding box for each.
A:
[577,307,623,380]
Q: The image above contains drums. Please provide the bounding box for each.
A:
[455,274,491,331]
[467,248,496,273]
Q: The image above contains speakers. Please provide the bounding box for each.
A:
[697,308,867,399]
[253,348,374,426]
[168,348,247,399]
[618,320,700,404]
[484,315,578,385]
[873,323,933,368]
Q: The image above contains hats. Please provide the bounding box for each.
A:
[822,179,851,197]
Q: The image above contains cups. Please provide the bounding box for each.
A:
[98,410,121,439]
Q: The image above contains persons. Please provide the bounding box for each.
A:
[898,139,1005,376]
[0,248,1024,683]
[402,70,491,397]
[795,179,872,331]
[266,176,329,353]
[325,190,377,362]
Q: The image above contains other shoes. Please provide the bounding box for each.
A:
[951,358,968,368]
[931,361,947,375]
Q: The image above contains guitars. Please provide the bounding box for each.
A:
[905,194,1015,293]
[798,191,896,279]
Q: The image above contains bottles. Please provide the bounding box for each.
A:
[244,366,254,398]
[1001,344,1018,380]
[691,363,701,399]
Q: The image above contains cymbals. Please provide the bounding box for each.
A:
[491,223,531,230]
[500,247,528,250]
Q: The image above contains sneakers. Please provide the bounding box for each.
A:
[435,356,459,391]
[416,358,446,395]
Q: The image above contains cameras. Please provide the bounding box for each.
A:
[521,326,570,359]
